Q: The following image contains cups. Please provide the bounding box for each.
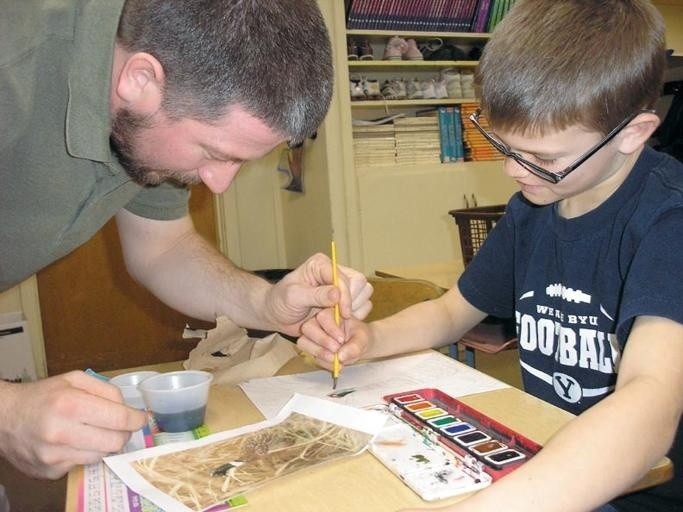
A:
[105,371,212,431]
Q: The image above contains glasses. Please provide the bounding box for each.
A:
[469,107,650,185]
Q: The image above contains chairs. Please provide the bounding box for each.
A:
[450,321,518,370]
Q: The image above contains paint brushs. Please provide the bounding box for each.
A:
[394,413,479,474]
[331,241,340,389]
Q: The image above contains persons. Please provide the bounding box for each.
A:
[0,0,375,512]
[296,0,683,512]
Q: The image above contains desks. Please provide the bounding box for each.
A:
[65,333,674,512]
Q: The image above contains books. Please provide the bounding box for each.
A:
[352,103,507,169]
[347,0,516,33]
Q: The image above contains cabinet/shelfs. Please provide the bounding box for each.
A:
[277,1,519,287]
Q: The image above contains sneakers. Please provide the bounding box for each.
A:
[348,35,482,99]
